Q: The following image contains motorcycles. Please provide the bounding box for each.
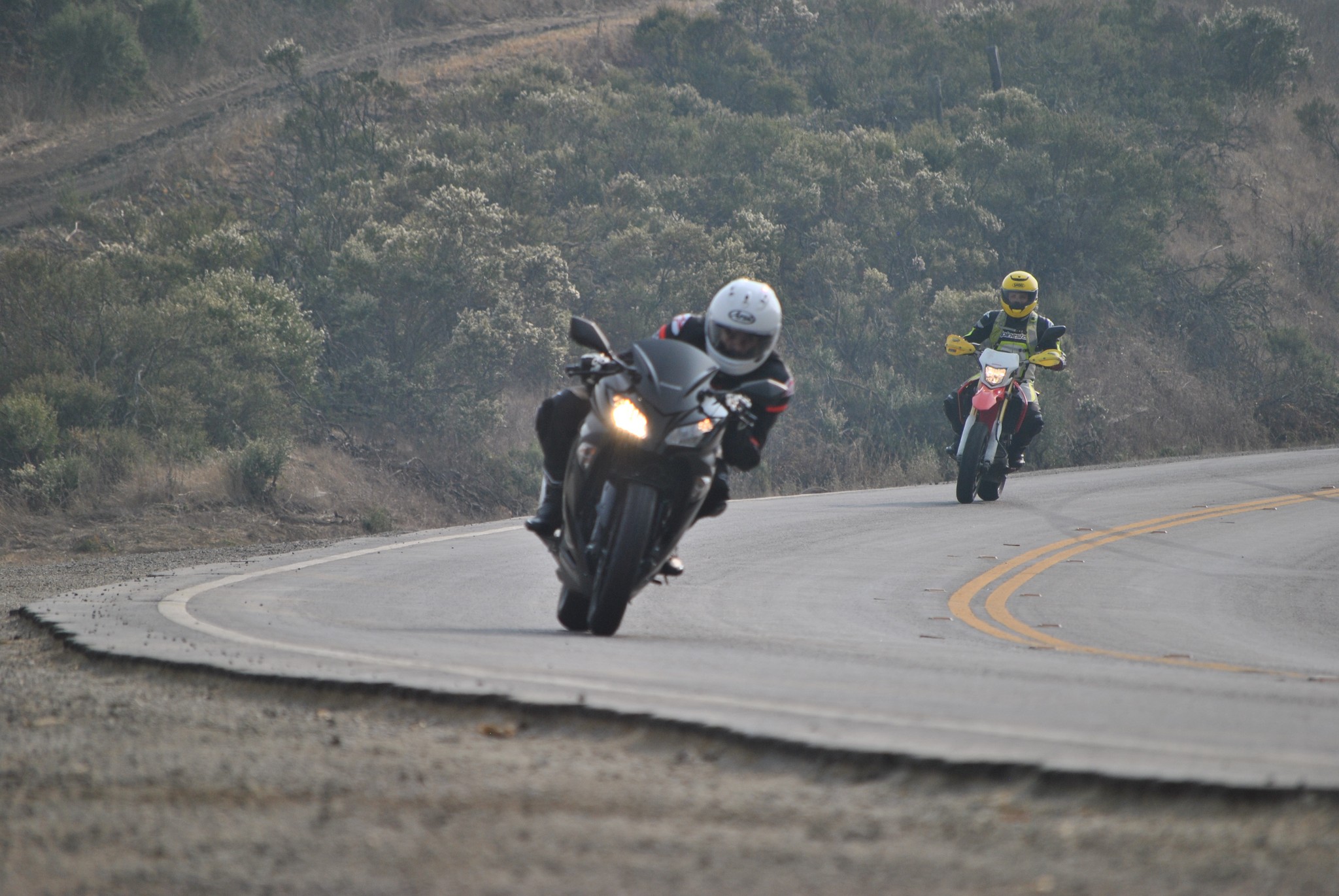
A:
[525,317,791,636]
[956,326,1067,504]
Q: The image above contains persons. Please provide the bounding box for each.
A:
[942,269,1066,464]
[523,276,794,575]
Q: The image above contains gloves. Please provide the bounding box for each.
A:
[719,392,753,432]
[581,352,611,384]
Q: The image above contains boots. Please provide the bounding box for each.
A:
[944,432,961,455]
[525,467,565,536]
[661,556,684,575]
[1008,440,1028,467]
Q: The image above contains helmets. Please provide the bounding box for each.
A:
[1000,271,1039,318]
[703,276,783,375]
